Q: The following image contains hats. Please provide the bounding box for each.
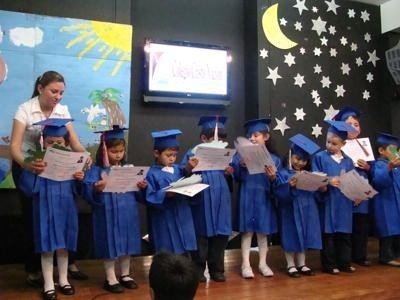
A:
[289,134,321,170]
[151,129,184,149]
[94,128,128,167]
[324,105,361,139]
[33,118,73,152]
[377,132,400,147]
[244,118,271,135]
[198,116,226,141]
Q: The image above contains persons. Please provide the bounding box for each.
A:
[270,133,330,279]
[8,69,95,290]
[82,123,148,295]
[230,116,286,280]
[331,105,376,274]
[369,129,400,267]
[147,248,200,300]
[16,117,97,300]
[140,127,199,265]
[181,114,236,284]
[310,117,363,276]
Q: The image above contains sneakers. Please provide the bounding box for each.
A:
[324,261,400,274]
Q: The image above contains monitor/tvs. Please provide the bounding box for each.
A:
[143,38,231,107]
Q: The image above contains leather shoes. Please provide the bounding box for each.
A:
[29,270,87,300]
[242,267,274,278]
[104,275,136,292]
[200,272,224,283]
[288,266,313,277]
